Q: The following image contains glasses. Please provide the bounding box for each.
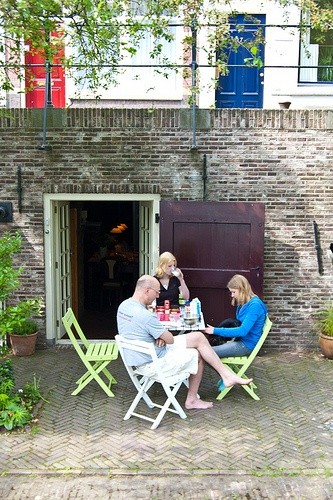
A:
[147,287,161,295]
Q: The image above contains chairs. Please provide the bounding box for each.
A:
[61,308,118,398]
[115,335,190,429]
[216,316,272,400]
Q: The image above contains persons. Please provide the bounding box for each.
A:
[117,275,254,410]
[202,275,268,393]
[151,252,189,308]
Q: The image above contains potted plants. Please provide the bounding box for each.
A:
[8,318,38,357]
[313,302,333,360]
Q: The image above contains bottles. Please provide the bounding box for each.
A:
[147,294,201,323]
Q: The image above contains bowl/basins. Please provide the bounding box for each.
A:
[184,317,197,325]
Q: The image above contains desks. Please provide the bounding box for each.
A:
[154,312,205,399]
